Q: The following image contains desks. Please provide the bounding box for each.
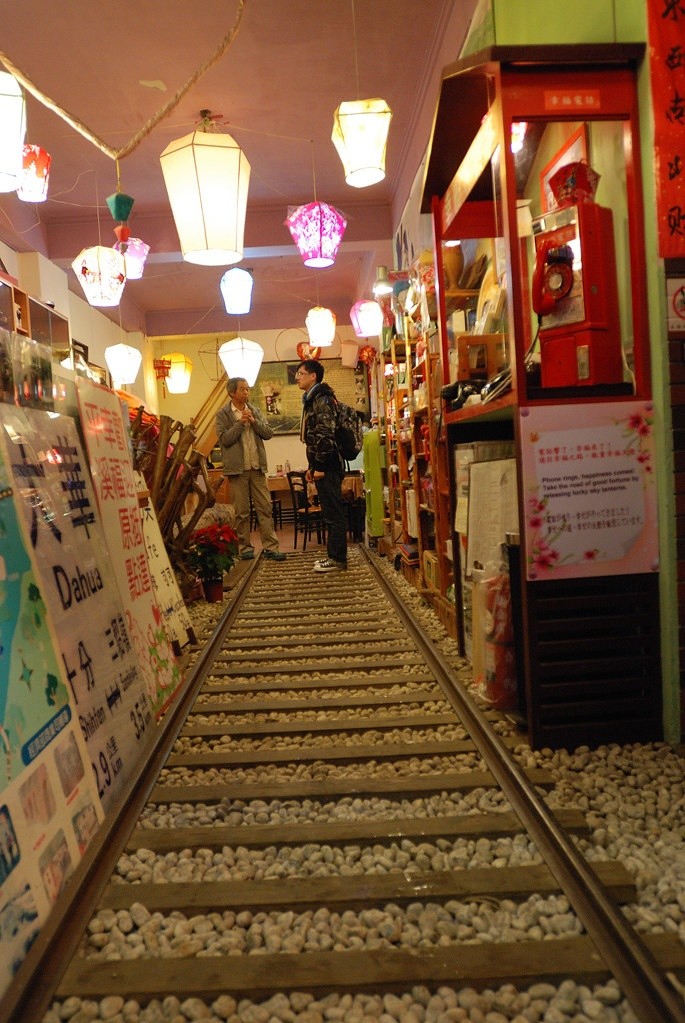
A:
[310,474,363,544]
[266,476,305,532]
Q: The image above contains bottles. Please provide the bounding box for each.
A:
[285,460,290,471]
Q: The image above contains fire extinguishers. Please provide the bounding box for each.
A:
[482,532,520,712]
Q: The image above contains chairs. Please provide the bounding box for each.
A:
[248,480,282,533]
[285,472,326,551]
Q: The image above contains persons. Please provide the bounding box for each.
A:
[214,378,286,561]
[295,360,368,572]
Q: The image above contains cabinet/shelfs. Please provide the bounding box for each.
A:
[0,41,664,750]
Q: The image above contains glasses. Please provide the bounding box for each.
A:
[294,372,312,378]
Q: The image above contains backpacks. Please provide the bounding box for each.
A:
[334,397,363,476]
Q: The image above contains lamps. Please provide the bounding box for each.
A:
[304,270,338,347]
[158,110,251,267]
[330,0,394,188]
[161,351,193,395]
[219,264,255,315]
[113,236,152,280]
[349,300,384,338]
[217,336,265,388]
[369,262,411,298]
[283,133,349,268]
[0,70,29,195]
[104,303,144,383]
[72,174,127,305]
[16,144,52,204]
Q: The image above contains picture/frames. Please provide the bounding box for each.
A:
[210,450,221,462]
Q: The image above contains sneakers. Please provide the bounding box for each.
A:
[313,557,328,565]
[314,560,346,571]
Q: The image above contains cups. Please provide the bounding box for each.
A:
[277,465,282,473]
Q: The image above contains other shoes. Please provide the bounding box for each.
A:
[263,548,286,560]
[241,550,254,560]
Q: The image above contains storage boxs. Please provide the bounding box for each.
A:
[422,551,442,588]
[455,441,514,498]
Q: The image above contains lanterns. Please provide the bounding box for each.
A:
[220,267,253,314]
[0,70,52,203]
[104,344,143,384]
[349,300,384,338]
[161,131,251,266]
[219,338,264,387]
[71,237,151,307]
[330,98,392,189]
[304,308,337,347]
[153,358,171,399]
[161,352,193,393]
[284,201,347,269]
